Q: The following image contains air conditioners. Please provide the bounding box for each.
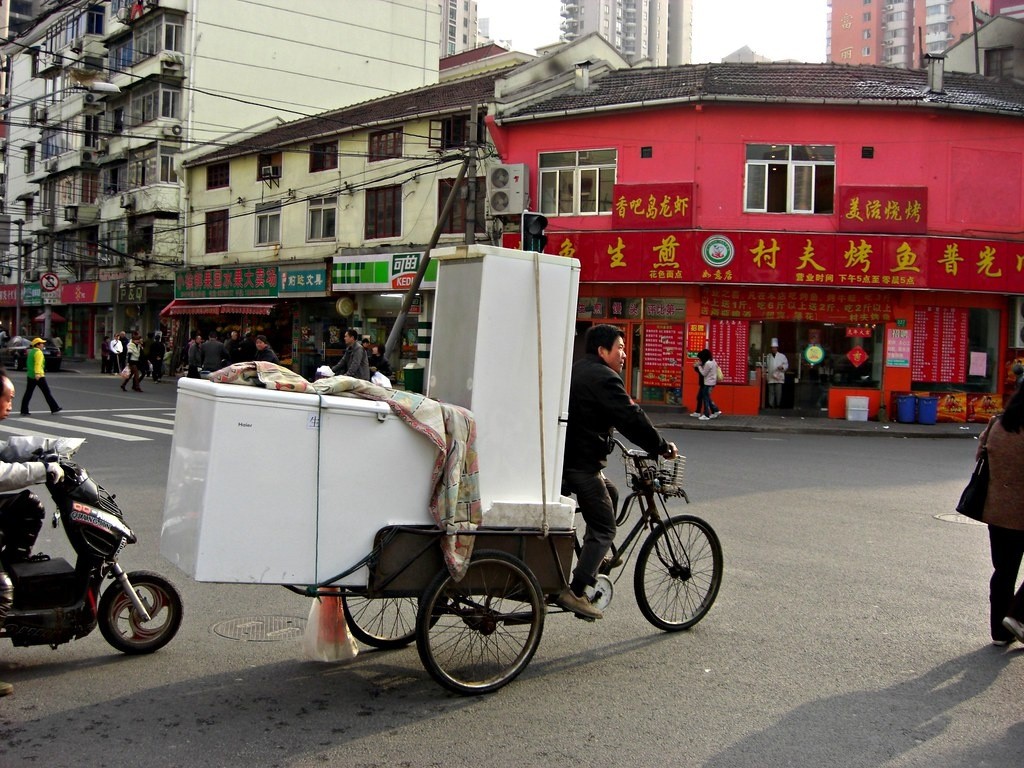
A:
[120,193,135,208]
[79,147,97,164]
[44,160,54,172]
[161,122,185,138]
[487,163,530,217]
[118,8,128,20]
[36,109,45,120]
[42,214,48,225]
[52,52,61,63]
[95,138,106,151]
[262,165,280,179]
[161,62,181,70]
[70,38,81,50]
[83,94,101,105]
[483,196,492,220]
[64,208,75,221]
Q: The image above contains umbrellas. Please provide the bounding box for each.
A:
[33,311,65,323]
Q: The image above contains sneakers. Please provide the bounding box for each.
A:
[709,410,721,418]
[603,555,622,568]
[698,414,710,421]
[1001,617,1024,644]
[689,411,701,418]
[557,585,604,620]
[991,637,1017,645]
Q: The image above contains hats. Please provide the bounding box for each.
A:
[770,338,778,348]
[254,334,268,343]
[30,337,46,347]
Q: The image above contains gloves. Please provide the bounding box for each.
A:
[47,461,64,484]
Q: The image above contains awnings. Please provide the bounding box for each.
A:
[159,300,276,316]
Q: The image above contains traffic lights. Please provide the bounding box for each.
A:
[522,213,548,253]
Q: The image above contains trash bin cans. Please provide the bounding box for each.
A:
[897,395,916,423]
[918,397,937,425]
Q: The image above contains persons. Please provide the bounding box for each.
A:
[1012,365,1024,389]
[100,331,173,392]
[750,344,755,349]
[187,331,281,379]
[556,325,678,618]
[0,329,8,349]
[0,369,66,696]
[690,349,721,420]
[766,338,788,409]
[332,329,392,382]
[21,338,62,416]
[974,379,1024,646]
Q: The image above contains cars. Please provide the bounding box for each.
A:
[0,335,62,372]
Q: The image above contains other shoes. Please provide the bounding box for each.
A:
[155,379,160,384]
[50,407,61,413]
[20,412,32,416]
[120,385,127,391]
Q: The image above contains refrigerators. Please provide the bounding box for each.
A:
[425,247,580,510]
[155,377,443,583]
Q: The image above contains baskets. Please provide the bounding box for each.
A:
[121,364,131,379]
[371,372,393,391]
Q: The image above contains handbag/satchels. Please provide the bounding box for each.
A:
[717,366,723,380]
[955,415,1002,522]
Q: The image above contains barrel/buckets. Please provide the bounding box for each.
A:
[896,396,917,422]
[918,397,937,425]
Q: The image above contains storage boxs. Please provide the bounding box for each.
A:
[845,408,869,422]
[845,395,869,409]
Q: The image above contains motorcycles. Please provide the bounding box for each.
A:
[0,446,186,654]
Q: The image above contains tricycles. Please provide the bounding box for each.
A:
[282,438,724,694]
